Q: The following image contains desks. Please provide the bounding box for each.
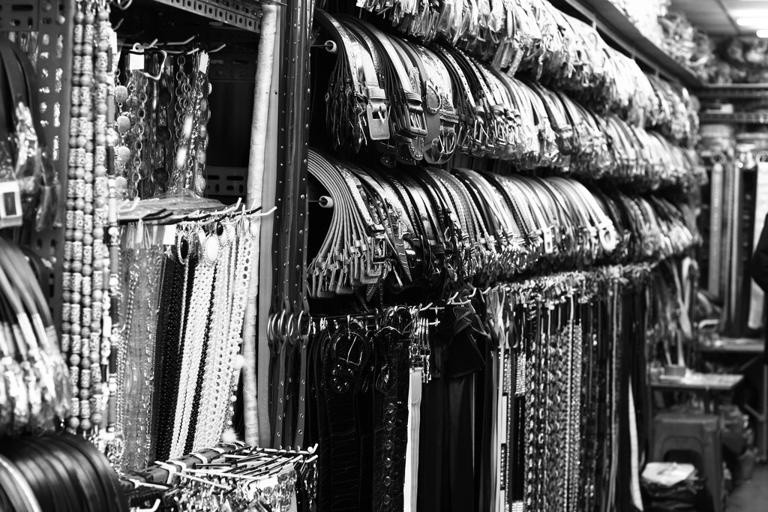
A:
[647,373,743,414]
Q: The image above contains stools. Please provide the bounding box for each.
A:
[649,406,723,512]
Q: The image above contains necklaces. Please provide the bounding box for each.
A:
[65,2,264,483]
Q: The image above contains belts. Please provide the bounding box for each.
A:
[306,151,705,311]
[309,263,695,512]
[314,0,697,192]
